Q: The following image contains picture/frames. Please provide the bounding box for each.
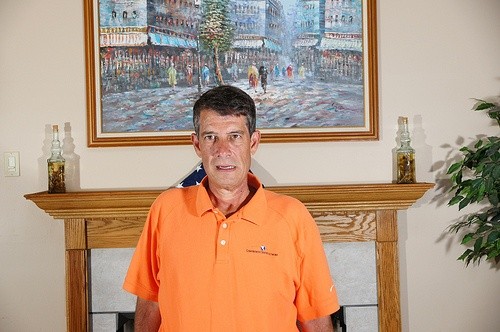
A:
[83,0,379,147]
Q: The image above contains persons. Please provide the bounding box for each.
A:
[121,86,340,332]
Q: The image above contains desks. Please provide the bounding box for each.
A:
[24,183,436,331]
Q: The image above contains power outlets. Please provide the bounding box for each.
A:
[4,152,20,177]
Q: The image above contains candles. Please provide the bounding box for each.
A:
[52,125,59,142]
[402,117,408,132]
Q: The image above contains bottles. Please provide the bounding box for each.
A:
[47,125,66,193]
[397,118,416,184]
